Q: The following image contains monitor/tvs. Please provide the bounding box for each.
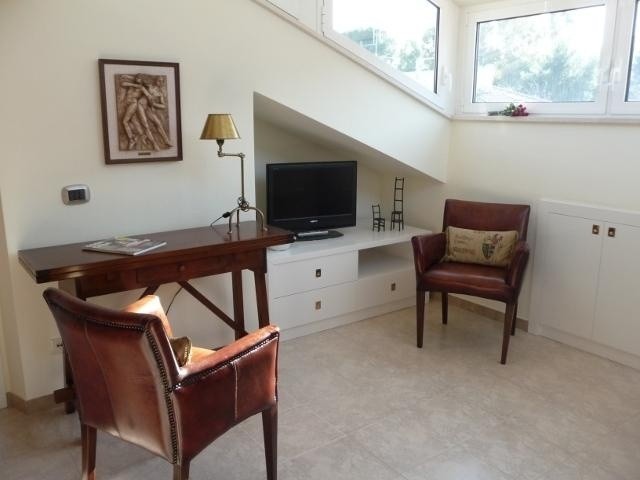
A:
[264,160,359,243]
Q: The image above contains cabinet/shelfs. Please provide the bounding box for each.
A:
[264,221,433,343]
[529,196,640,370]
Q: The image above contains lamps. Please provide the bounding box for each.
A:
[201,114,269,240]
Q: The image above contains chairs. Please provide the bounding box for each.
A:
[390,177,404,231]
[44,288,280,480]
[412,198,530,364]
[372,204,384,233]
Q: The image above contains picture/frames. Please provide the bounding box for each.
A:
[98,59,183,164]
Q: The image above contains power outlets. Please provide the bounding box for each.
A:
[50,338,66,356]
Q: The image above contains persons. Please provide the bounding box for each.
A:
[138,75,173,148]
[121,73,160,151]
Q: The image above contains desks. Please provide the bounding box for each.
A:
[19,220,299,410]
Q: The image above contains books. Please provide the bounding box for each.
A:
[82,236,167,256]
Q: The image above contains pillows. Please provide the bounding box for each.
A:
[169,336,191,365]
[442,226,518,267]
